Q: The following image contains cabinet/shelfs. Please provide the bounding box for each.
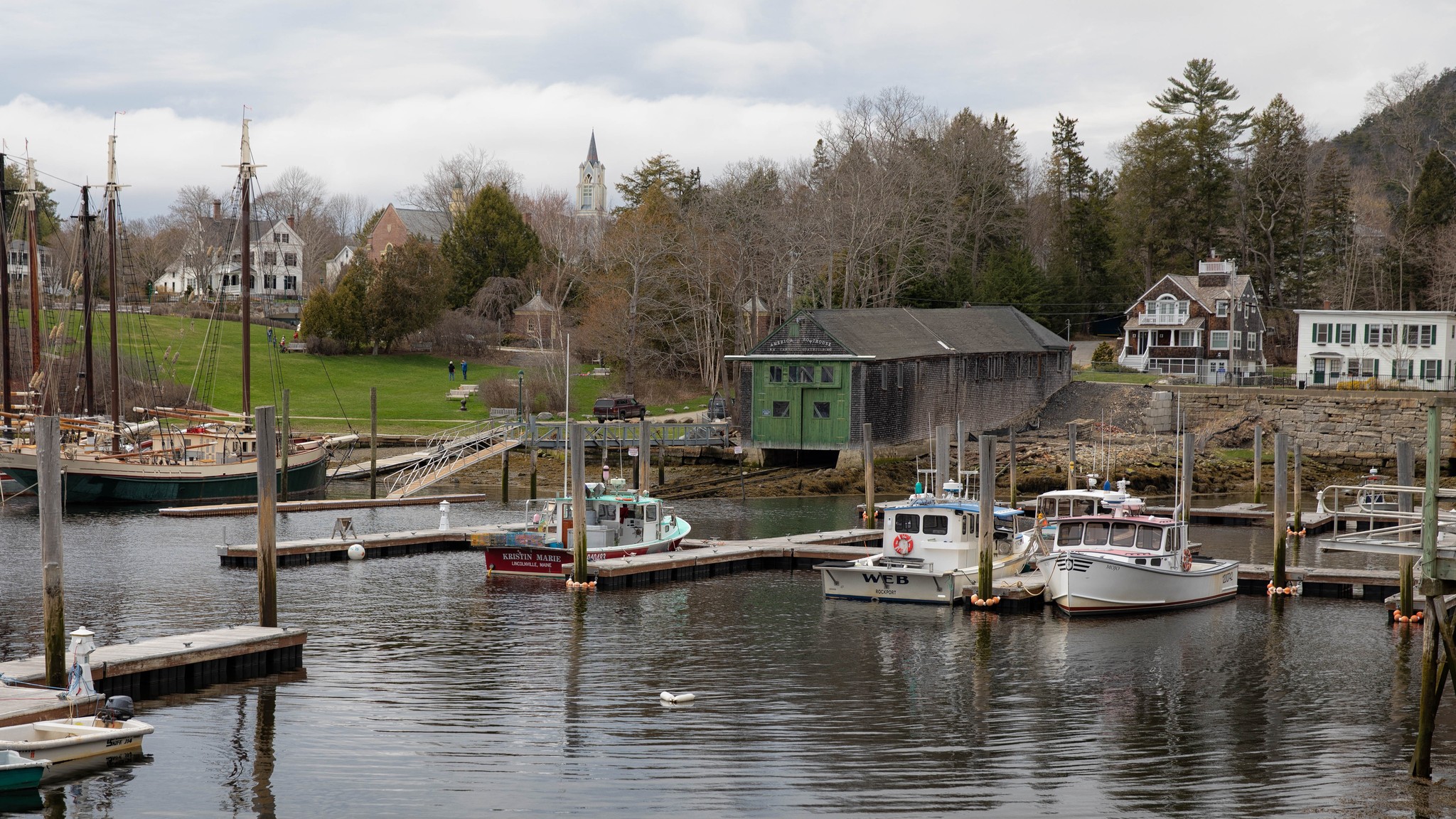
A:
[567,528,614,548]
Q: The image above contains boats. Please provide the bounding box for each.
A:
[1344,465,1399,515]
[812,411,1038,605]
[0,695,155,793]
[1033,390,1244,617]
[471,407,692,580]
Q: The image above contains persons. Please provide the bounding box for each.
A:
[448,361,455,381]
[460,360,467,380]
[296,321,301,331]
[612,504,628,524]
[279,335,290,352]
[191,320,195,331]
[273,335,277,348]
[267,327,273,344]
[570,371,590,376]
[293,331,298,341]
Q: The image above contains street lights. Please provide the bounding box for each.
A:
[517,370,524,428]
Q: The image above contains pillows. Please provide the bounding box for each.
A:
[458,386,463,390]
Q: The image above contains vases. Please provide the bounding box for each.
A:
[189,457,194,461]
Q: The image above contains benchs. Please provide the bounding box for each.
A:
[53,302,151,315]
[410,342,433,353]
[287,342,307,354]
[456,384,479,396]
[445,389,470,402]
[589,367,611,379]
[504,379,525,388]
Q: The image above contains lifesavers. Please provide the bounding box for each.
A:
[1181,548,1192,570]
[893,534,913,554]
[616,497,633,501]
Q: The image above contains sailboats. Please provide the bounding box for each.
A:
[0,101,360,511]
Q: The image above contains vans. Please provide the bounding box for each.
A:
[707,396,735,419]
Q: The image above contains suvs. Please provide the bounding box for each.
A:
[593,394,647,424]
